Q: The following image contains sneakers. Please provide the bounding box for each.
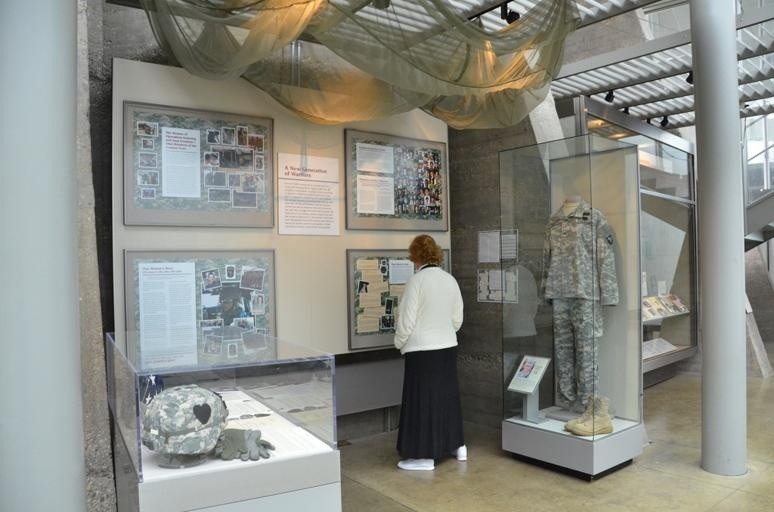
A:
[397,458,434,470]
[452,445,467,460]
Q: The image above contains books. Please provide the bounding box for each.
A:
[641,292,690,322]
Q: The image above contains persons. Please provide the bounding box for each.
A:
[540,194,621,423]
[391,143,444,221]
[380,290,399,331]
[135,120,264,207]
[201,264,266,357]
[358,281,368,293]
[518,360,534,378]
[392,234,469,472]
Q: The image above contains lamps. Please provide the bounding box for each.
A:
[498,2,519,25]
[685,71,693,86]
[602,89,670,131]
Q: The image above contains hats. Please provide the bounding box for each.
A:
[141,384,228,456]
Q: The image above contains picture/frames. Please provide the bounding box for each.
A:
[120,98,275,230]
[342,127,449,234]
[345,248,452,351]
[122,246,278,376]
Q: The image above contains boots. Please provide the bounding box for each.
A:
[573,399,612,437]
[565,396,596,432]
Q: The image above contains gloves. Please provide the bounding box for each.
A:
[236,430,275,461]
[221,429,248,461]
